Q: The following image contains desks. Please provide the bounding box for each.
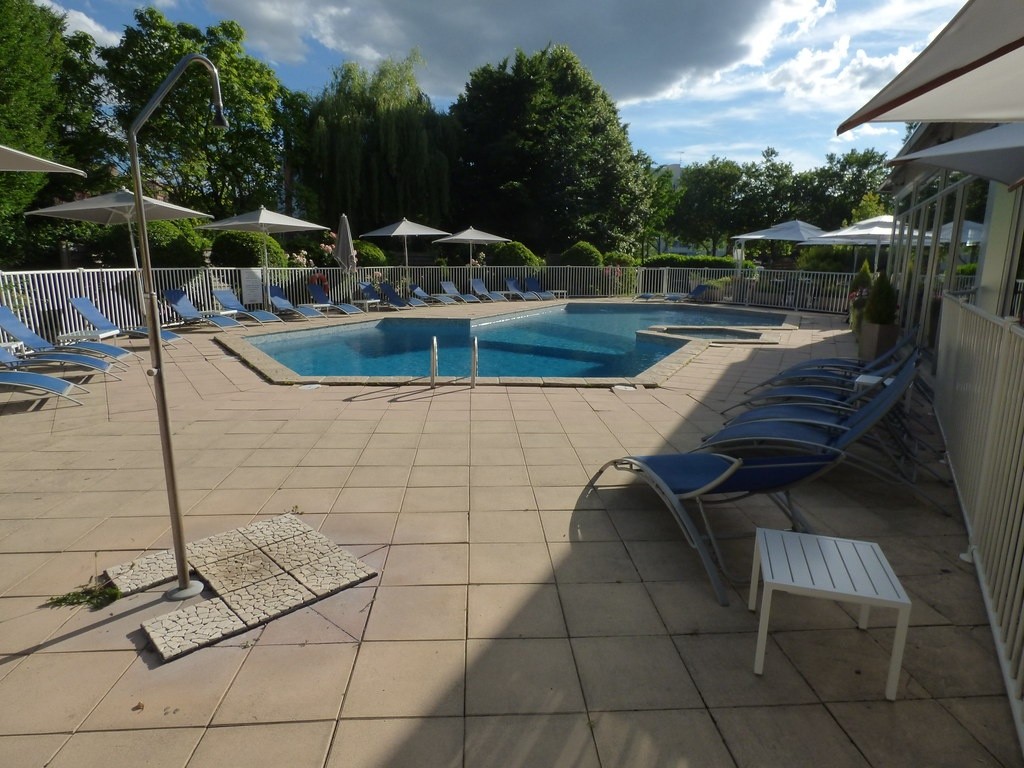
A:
[199,310,237,319]
[432,294,456,299]
[748,527,912,701]
[490,291,516,301]
[854,374,913,418]
[0,341,28,372]
[58,329,120,344]
[548,290,567,298]
[297,304,330,317]
[352,299,380,313]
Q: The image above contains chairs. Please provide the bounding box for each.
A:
[600,322,923,607]
[264,285,369,322]
[68,298,192,349]
[211,290,287,327]
[439,277,510,304]
[162,289,248,334]
[0,346,127,381]
[632,285,708,305]
[0,306,145,368]
[360,282,460,312]
[504,275,556,302]
[0,372,89,406]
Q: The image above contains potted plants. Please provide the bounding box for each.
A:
[858,270,900,368]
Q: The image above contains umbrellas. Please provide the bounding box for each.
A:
[794,213,936,273]
[927,216,987,244]
[359,217,453,297]
[332,213,358,303]
[192,205,331,313]
[432,226,512,294]
[836,0,1024,138]
[0,146,87,179]
[731,220,828,276]
[882,122,1024,193]
[23,185,214,316]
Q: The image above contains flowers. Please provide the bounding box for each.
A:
[850,289,868,305]
